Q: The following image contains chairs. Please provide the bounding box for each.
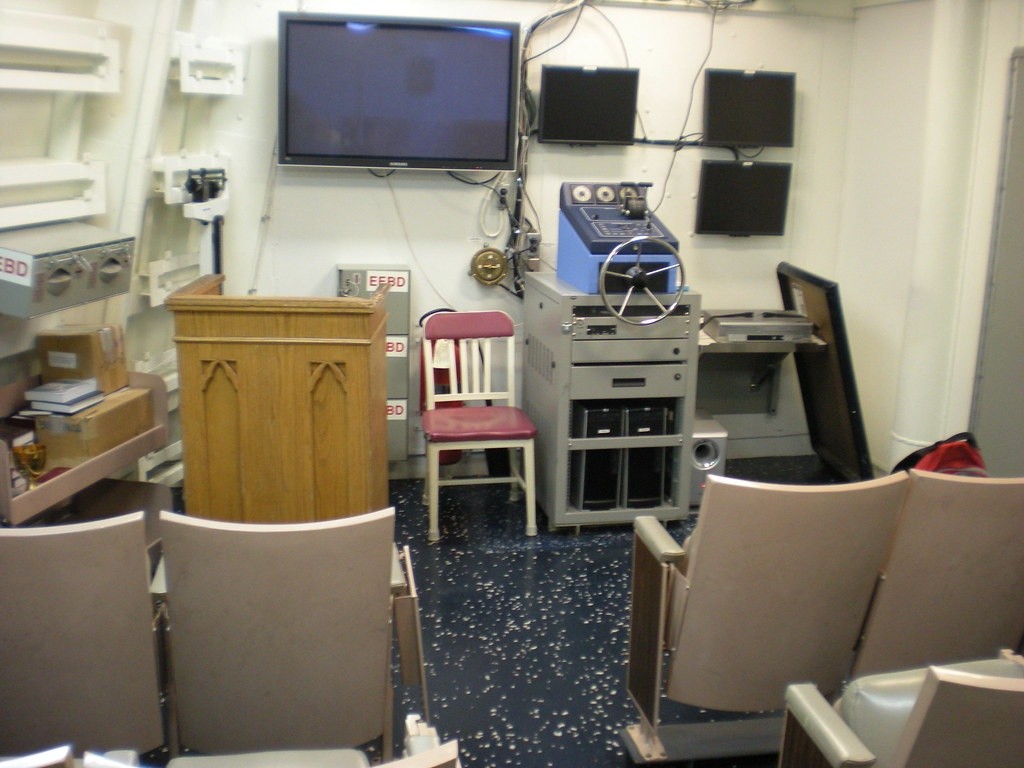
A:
[612,459,1023,768]
[0,501,462,768]
[421,311,537,542]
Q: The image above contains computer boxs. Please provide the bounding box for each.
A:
[571,400,668,510]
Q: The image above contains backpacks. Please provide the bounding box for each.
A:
[891,432,987,478]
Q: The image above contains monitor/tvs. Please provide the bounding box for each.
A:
[538,64,639,145]
[702,68,796,148]
[694,159,792,238]
[276,11,520,172]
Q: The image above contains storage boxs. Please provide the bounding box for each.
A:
[38,320,131,397]
[33,384,153,472]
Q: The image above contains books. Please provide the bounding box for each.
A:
[7,376,106,421]
